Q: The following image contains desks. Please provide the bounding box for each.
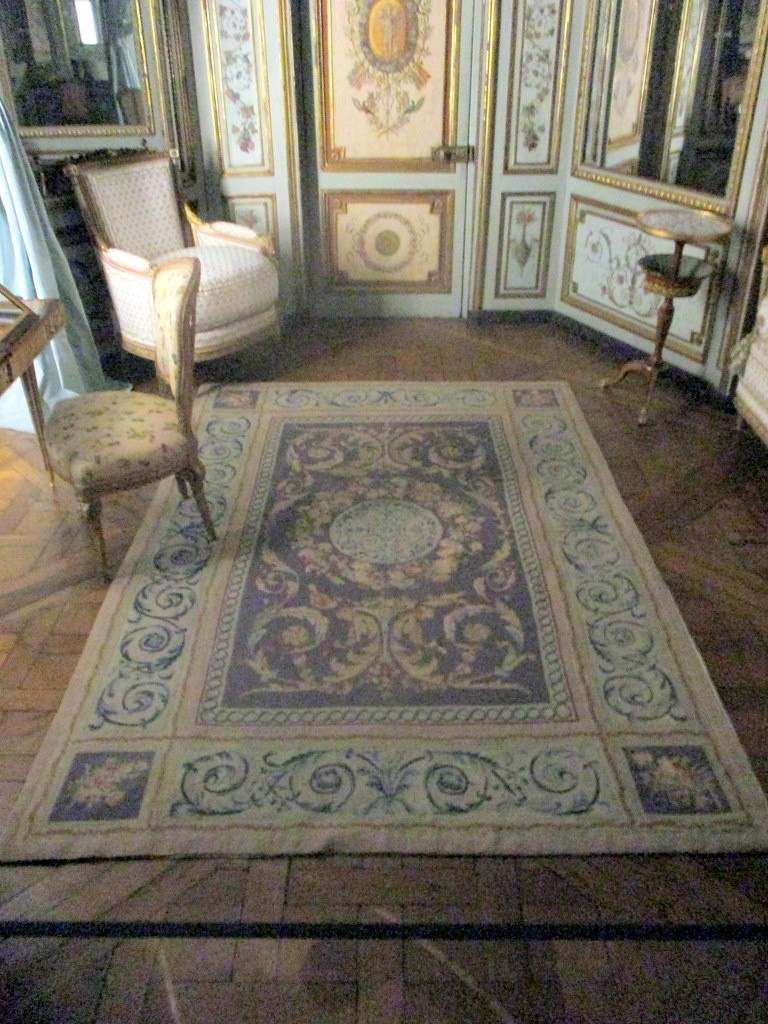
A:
[0,284,70,482]
[591,207,732,428]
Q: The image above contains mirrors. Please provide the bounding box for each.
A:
[565,0,768,220]
[0,0,156,138]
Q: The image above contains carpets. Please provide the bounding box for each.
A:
[0,378,768,860]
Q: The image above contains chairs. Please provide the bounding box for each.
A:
[61,150,284,379]
[42,252,222,585]
